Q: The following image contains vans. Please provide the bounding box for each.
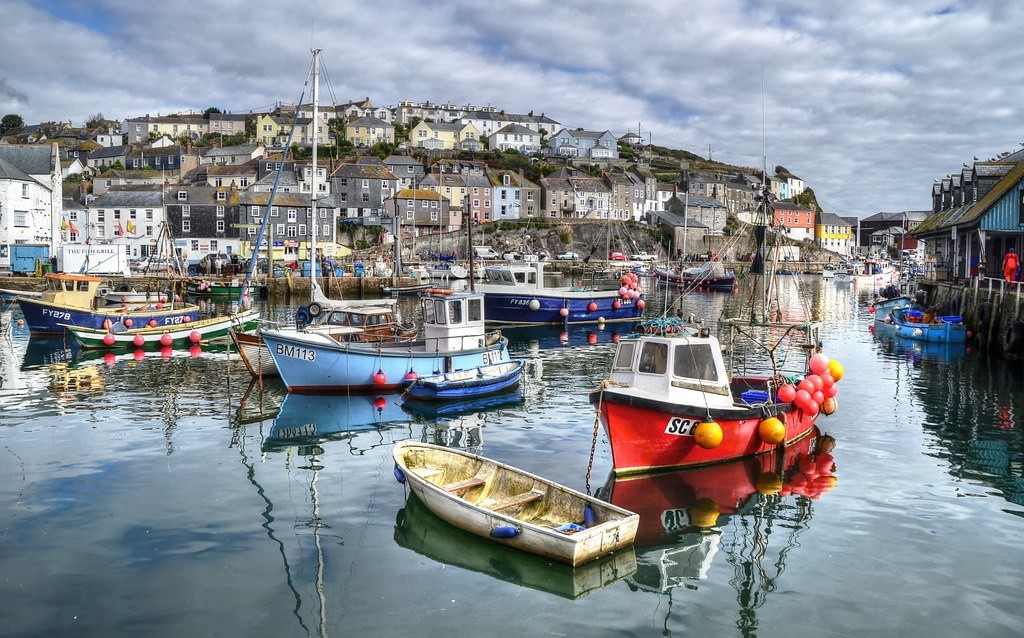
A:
[473,247,500,260]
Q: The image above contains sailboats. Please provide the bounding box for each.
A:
[233,377,328,638]
[873,216,920,327]
[229,47,418,382]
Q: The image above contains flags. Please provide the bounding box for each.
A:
[126,220,137,234]
[70,220,79,234]
[118,220,124,236]
[60,216,72,230]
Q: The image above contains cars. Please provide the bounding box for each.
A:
[131,253,174,273]
[245,256,282,274]
[610,252,628,261]
[556,251,580,261]
[189,253,240,274]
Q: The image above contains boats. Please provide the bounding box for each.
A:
[459,264,644,331]
[776,268,797,275]
[587,425,822,595]
[821,265,836,278]
[406,359,525,398]
[648,259,737,292]
[389,493,639,600]
[834,257,898,288]
[895,309,969,344]
[259,390,523,450]
[394,440,642,567]
[17,160,264,347]
[20,329,259,412]
[403,389,522,422]
[583,66,823,476]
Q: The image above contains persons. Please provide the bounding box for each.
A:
[922,302,938,326]
[203,248,259,278]
[51,255,58,273]
[1001,247,1021,289]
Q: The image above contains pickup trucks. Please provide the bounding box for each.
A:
[630,252,659,261]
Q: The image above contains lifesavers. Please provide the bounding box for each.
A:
[295,310,309,324]
[434,289,453,295]
